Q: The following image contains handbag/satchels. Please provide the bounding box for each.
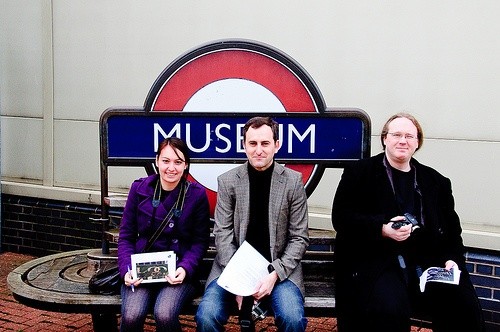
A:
[90,263,122,294]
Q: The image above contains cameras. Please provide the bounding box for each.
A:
[393,212,421,236]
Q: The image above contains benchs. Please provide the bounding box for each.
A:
[7,247,433,332]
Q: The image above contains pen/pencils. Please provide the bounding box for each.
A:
[128,265,135,293]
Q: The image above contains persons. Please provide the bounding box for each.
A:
[195,117,309,332]
[331,112,485,332]
[117,135,211,332]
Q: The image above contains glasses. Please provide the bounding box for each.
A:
[387,132,419,140]
